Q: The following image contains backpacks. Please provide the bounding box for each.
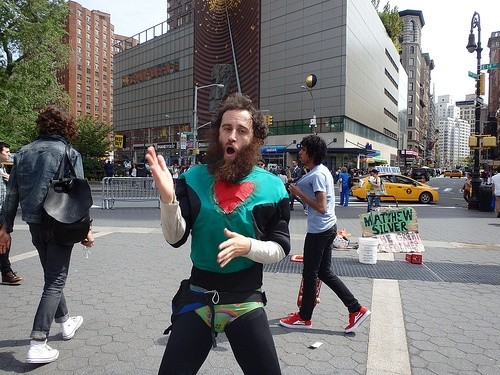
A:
[37,144,93,245]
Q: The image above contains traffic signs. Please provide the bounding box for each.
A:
[468,70,478,80]
[481,63,498,70]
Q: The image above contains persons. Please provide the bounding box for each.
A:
[0,142,23,284]
[169,163,189,188]
[338,166,351,207]
[145,93,291,375]
[461,166,500,218]
[405,163,412,178]
[129,163,138,187]
[267,155,366,187]
[367,169,383,214]
[288,159,307,210]
[420,172,427,184]
[256,159,267,169]
[105,161,114,183]
[280,135,371,334]
[0,105,95,364]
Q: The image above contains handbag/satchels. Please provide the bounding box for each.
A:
[372,184,388,195]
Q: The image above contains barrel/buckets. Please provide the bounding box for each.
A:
[358,237,378,264]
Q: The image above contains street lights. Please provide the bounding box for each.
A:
[300,86,317,135]
[466,11,484,210]
[193,84,224,166]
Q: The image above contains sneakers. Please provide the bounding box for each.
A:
[344,306,372,333]
[279,312,312,329]
[25,339,59,363]
[60,315,83,340]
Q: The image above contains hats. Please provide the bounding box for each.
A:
[258,159,265,164]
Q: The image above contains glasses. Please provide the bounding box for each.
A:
[257,164,263,166]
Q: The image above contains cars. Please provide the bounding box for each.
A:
[444,171,451,177]
[450,169,461,178]
[412,168,445,182]
[129,163,150,177]
[351,172,439,203]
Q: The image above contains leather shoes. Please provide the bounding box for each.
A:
[2,270,23,283]
